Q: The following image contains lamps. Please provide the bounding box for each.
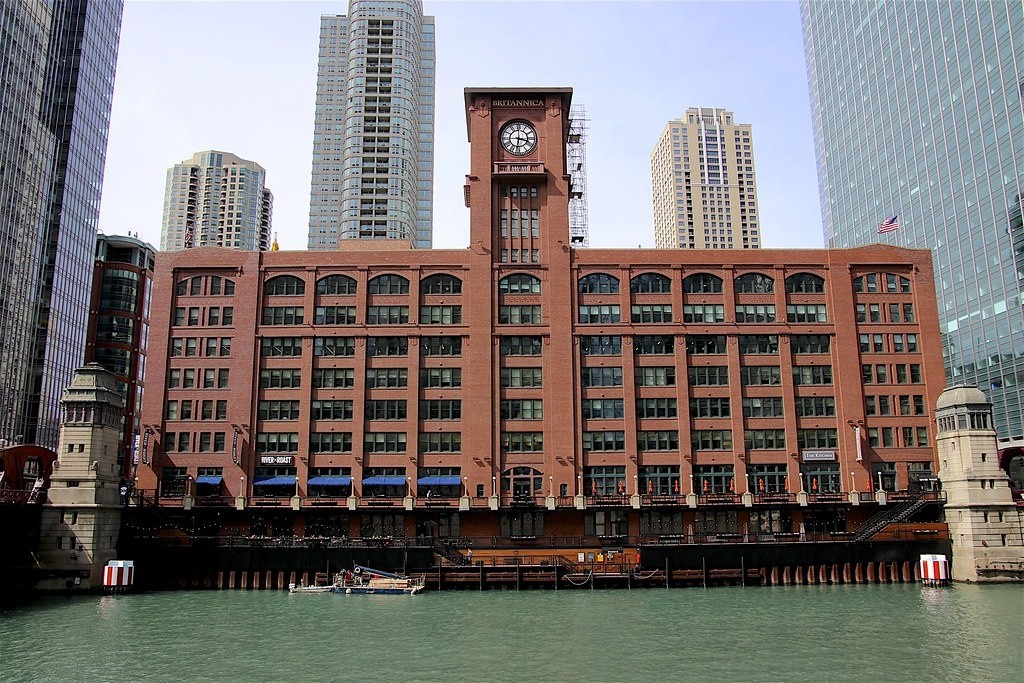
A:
[737,453,745,459]
[408,456,417,460]
[300,456,307,460]
[354,456,363,461]
[630,455,638,461]
[683,455,691,459]
[556,455,574,461]
[474,456,491,461]
[848,419,864,426]
[790,453,799,456]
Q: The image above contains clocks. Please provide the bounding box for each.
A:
[500,120,536,155]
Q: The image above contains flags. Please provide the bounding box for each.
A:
[878,215,899,234]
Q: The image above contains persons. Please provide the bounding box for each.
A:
[427,490,433,499]
[346,570,351,583]
[280,535,393,546]
[466,548,473,563]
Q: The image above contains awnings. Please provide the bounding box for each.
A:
[252,477,296,485]
[418,477,460,485]
[306,477,351,486]
[362,476,405,484]
[194,477,223,485]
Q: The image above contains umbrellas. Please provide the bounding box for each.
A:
[730,479,735,493]
[812,478,816,493]
[866,480,870,492]
[618,480,624,495]
[675,480,679,494]
[784,477,789,493]
[759,478,764,495]
[592,481,597,493]
[703,479,709,493]
[648,480,653,495]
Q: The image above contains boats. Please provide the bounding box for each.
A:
[288,583,333,594]
[332,560,427,595]
